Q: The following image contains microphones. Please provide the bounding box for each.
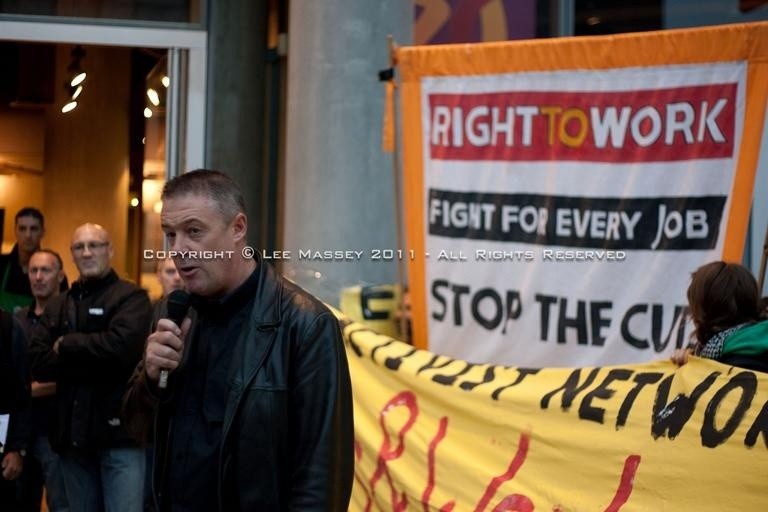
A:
[155,288,191,388]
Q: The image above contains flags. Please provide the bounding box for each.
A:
[400,21,768,370]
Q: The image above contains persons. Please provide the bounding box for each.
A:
[0,207,68,298]
[117,165,355,511]
[674,260,768,380]
[143,247,186,334]
[10,247,72,511]
[0,306,46,511]
[39,219,152,510]
